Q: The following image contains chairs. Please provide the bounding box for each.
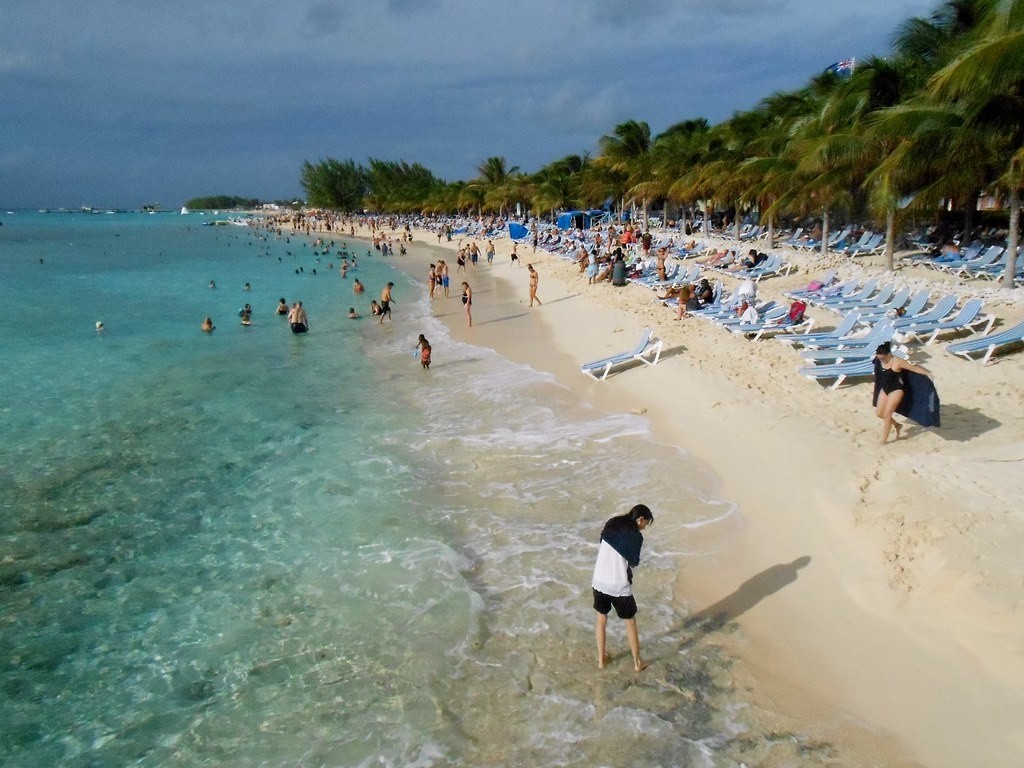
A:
[581,328,663,380]
[799,349,911,389]
[465,209,1024,335]
[775,316,858,349]
[800,327,895,365]
[949,322,1024,366]
[899,296,997,346]
[800,317,895,353]
[727,318,814,342]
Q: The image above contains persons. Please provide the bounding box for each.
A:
[592,504,655,671]
[95,203,803,371]
[872,341,934,447]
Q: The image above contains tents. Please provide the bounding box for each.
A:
[508,223,529,239]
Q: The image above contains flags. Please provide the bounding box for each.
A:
[825,57,856,82]
